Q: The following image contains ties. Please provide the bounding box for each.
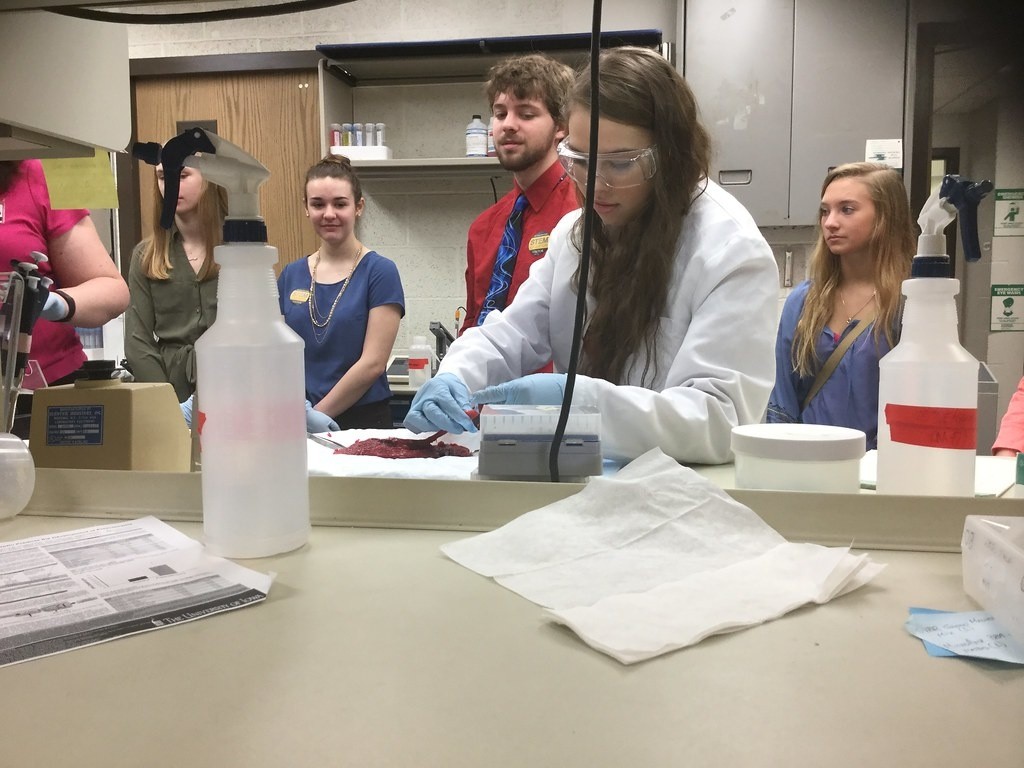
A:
[477,194,529,326]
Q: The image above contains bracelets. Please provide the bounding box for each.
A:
[48,290,75,322]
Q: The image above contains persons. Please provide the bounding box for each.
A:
[403,45,779,464]
[277,154,405,434]
[766,161,918,452]
[124,141,229,428]
[0,122,131,440]
[457,51,583,413]
[990,375,1024,457]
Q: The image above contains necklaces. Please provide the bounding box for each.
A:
[309,244,363,344]
[841,287,877,324]
[186,249,208,262]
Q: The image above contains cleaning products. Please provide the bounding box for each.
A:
[133,126,316,560]
[874,172,997,499]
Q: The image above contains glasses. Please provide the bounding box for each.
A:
[556,135,658,188]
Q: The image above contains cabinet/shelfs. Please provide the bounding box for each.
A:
[315,29,665,174]
[674,0,914,222]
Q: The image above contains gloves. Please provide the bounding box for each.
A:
[305,399,340,434]
[39,292,65,322]
[179,395,196,428]
[473,373,566,405]
[403,373,478,435]
